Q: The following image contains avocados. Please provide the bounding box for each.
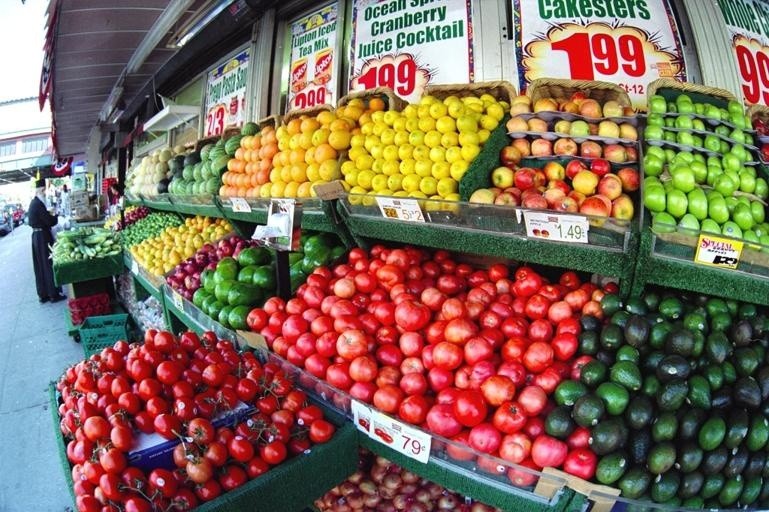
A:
[304,233,326,255]
[299,228,314,246]
[546,293,769,512]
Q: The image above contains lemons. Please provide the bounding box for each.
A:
[336,93,510,216]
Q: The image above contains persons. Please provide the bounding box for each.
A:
[27,179,67,303]
[111,184,123,205]
[61,184,68,209]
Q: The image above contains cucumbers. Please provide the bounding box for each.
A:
[57,227,122,261]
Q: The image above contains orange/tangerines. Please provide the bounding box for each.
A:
[119,210,182,249]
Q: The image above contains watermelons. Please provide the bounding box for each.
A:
[158,121,260,204]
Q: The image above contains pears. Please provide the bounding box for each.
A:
[131,216,233,277]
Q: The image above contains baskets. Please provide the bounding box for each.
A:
[78,312,132,360]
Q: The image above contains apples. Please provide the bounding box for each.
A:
[470,92,768,252]
[104,205,149,232]
[218,124,279,197]
[237,238,246,248]
[247,242,258,248]
[230,235,241,247]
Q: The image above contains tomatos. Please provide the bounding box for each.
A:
[55,245,620,512]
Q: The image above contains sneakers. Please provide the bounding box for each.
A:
[38,292,67,304]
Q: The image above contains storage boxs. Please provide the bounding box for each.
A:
[78,314,132,359]
[71,188,97,222]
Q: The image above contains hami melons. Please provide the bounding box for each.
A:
[129,144,194,201]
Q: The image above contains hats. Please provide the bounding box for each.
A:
[35,178,46,187]
